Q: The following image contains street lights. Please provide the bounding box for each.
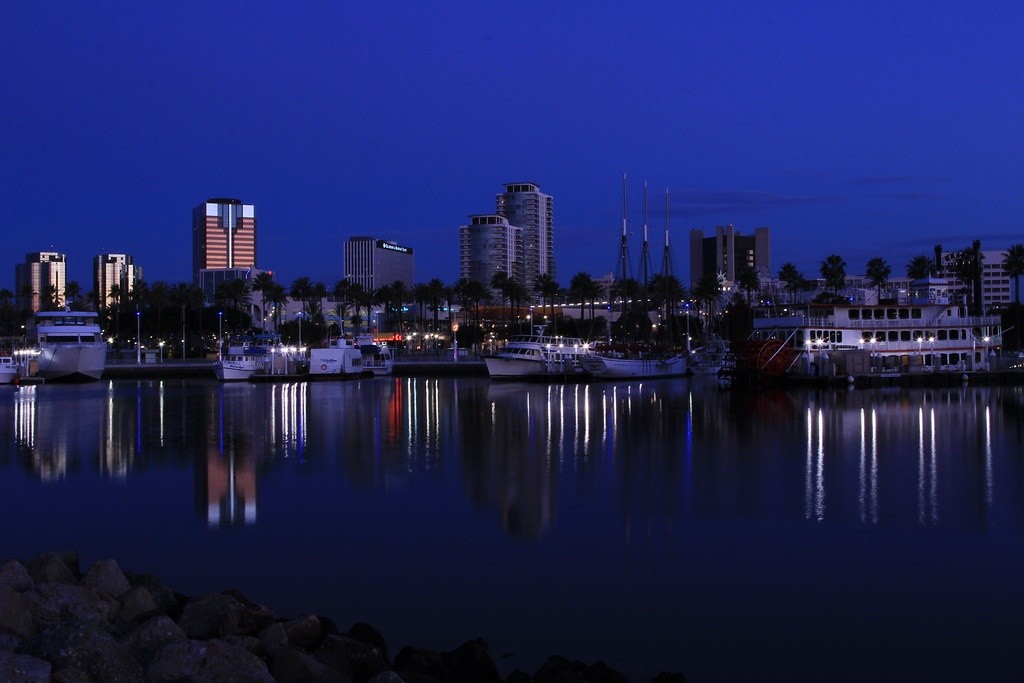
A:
[283,348,289,374]
[452,323,459,363]
[270,348,275,375]
[302,347,306,359]
[299,348,302,358]
[159,342,164,361]
[218,312,224,362]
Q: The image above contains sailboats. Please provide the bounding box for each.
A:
[576,170,705,379]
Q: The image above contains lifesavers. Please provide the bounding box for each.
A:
[320,364,328,371]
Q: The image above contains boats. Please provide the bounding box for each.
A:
[136,311,141,364]
[480,334,598,380]
[690,339,734,375]
[0,355,26,385]
[213,335,267,381]
[360,344,393,376]
[24,305,108,380]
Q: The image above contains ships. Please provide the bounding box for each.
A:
[743,302,1024,390]
